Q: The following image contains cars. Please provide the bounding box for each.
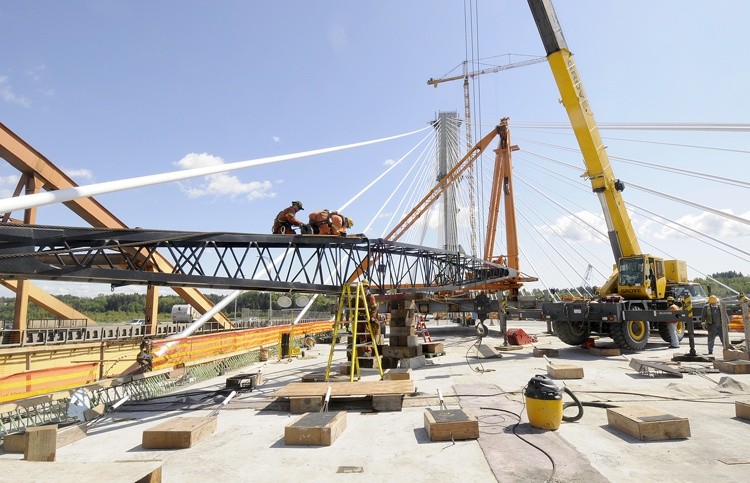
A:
[668,282,712,329]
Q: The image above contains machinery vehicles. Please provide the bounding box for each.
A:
[349,119,544,320]
[528,1,716,363]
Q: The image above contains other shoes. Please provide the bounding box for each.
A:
[669,345,680,348]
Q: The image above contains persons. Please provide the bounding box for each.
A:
[347,279,379,360]
[665,296,680,348]
[272,201,306,234]
[701,295,735,354]
[307,209,354,235]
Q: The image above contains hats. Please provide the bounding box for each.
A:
[362,279,370,286]
[708,296,719,304]
[666,297,674,301]
[345,217,354,230]
[292,200,304,210]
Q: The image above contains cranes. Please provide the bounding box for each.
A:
[427,54,549,263]
